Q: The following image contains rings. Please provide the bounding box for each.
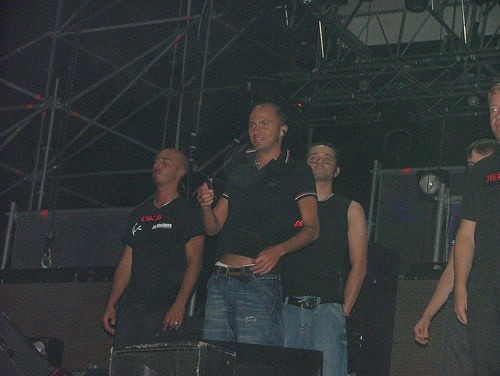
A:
[174,321,179,325]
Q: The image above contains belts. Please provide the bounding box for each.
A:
[282,296,337,310]
[212,267,263,284]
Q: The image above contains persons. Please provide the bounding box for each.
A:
[414,146,495,375]
[282,142,367,376]
[197,101,319,346]
[102,148,205,344]
[454,84,500,376]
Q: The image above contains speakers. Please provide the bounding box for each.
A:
[385,262,448,376]
[108,340,323,376]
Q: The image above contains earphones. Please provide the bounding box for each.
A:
[281,129,286,134]
